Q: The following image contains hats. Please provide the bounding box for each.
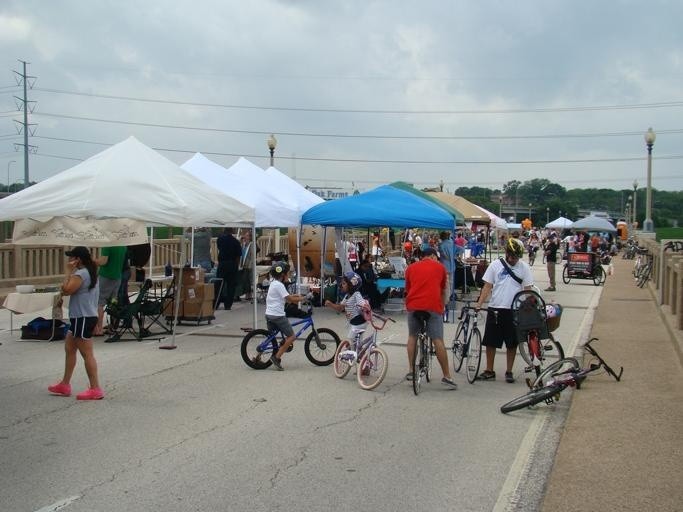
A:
[418,247,442,261]
[63,245,91,259]
[547,235,554,239]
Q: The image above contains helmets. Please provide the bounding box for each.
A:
[505,237,525,260]
[342,270,363,294]
[268,260,291,280]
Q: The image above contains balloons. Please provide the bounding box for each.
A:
[520,219,532,229]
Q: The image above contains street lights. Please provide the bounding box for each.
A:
[437,179,444,191]
[266,132,278,167]
[527,203,567,227]
[641,126,657,234]
[7,160,16,193]
[498,194,503,217]
[623,194,632,223]
[631,177,639,231]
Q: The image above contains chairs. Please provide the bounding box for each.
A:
[139,274,179,337]
[103,277,152,344]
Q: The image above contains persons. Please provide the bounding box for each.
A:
[184,226,211,284]
[253,261,308,371]
[48,246,104,400]
[92,246,131,336]
[212,226,242,310]
[232,231,261,302]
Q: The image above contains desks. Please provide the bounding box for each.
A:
[1,292,64,342]
[375,278,405,314]
[254,265,272,304]
[454,260,494,289]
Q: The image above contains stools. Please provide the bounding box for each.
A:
[460,265,472,294]
[256,282,270,304]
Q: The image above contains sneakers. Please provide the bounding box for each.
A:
[439,376,459,389]
[474,369,496,383]
[544,286,557,292]
[269,355,285,371]
[75,386,105,401]
[504,371,514,384]
[46,382,73,397]
[405,371,418,382]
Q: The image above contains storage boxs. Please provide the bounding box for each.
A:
[164,266,215,322]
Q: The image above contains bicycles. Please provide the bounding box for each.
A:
[511,287,568,393]
[542,251,562,265]
[237,291,327,372]
[332,303,397,395]
[408,309,432,397]
[526,245,536,268]
[620,238,653,291]
[448,306,488,386]
[497,337,623,416]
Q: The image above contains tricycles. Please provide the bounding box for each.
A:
[560,249,608,288]
